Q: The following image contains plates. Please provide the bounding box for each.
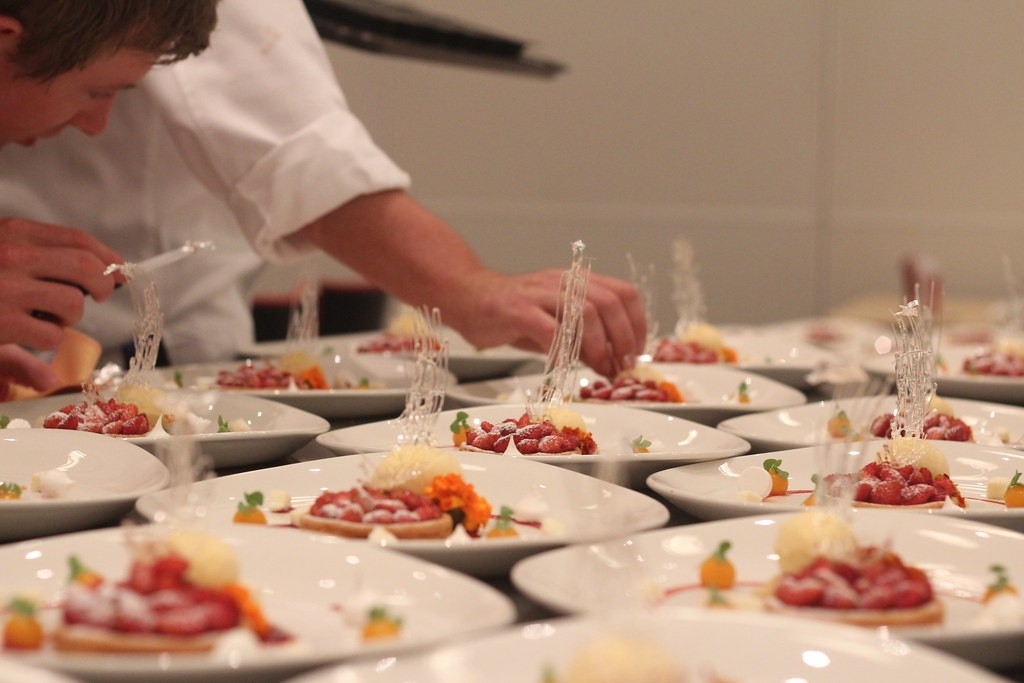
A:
[445,368,808,425]
[0,524,515,682]
[315,404,751,491]
[646,444,1024,529]
[135,451,669,577]
[298,613,1009,683]
[717,395,1024,451]
[632,451,638,456]
[512,509,1024,664]
[0,392,329,468]
[152,356,456,419]
[631,307,1024,400]
[0,427,168,545]
[235,340,533,379]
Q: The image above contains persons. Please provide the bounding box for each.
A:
[0,0,646,376]
[0,0,218,404]
